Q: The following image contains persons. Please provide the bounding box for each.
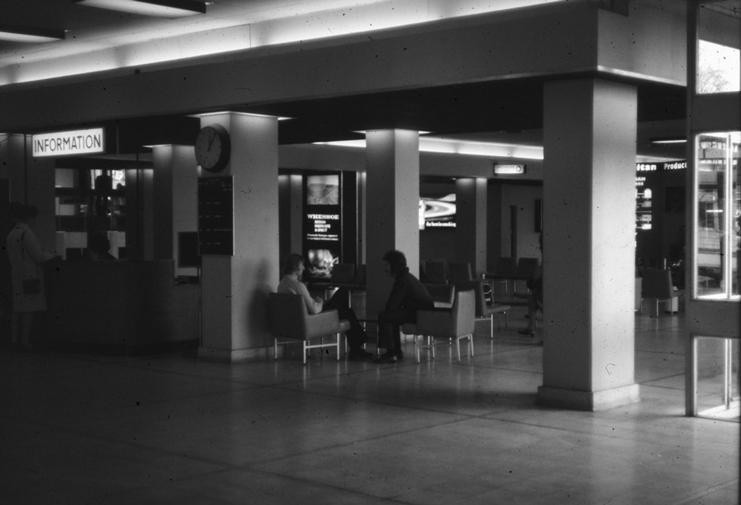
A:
[275,254,372,359]
[0,199,55,353]
[375,250,436,362]
[85,238,118,263]
[518,266,544,339]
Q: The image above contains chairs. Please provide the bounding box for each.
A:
[641,269,681,320]
[269,257,536,364]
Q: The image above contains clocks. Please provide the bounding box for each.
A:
[195,124,230,173]
